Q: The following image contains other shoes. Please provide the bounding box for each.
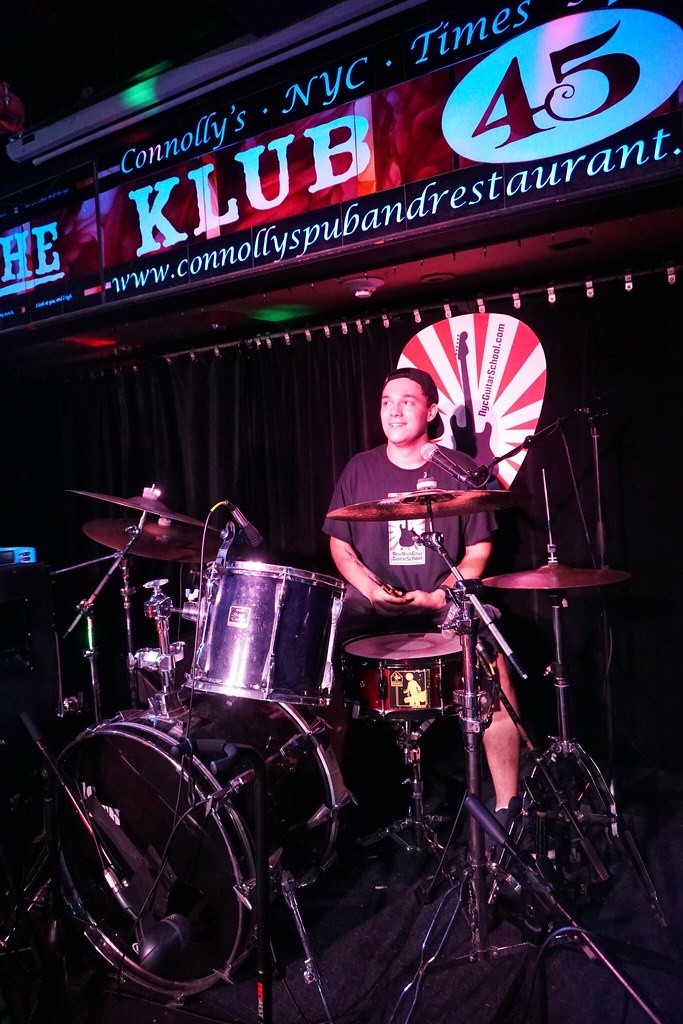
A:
[485,797,535,858]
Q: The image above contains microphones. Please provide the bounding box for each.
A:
[225,501,263,547]
[137,913,193,973]
[475,633,491,664]
[420,442,479,488]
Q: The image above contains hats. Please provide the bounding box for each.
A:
[383,368,444,440]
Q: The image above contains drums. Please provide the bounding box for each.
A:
[45,690,349,1000]
[184,559,347,706]
[341,629,481,722]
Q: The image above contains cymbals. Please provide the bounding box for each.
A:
[480,561,632,590]
[65,487,222,533]
[81,516,242,565]
[325,485,531,522]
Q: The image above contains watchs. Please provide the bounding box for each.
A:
[438,585,453,604]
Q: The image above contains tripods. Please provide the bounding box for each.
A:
[353,400,683,1024]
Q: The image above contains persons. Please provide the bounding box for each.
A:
[315,367,520,862]
